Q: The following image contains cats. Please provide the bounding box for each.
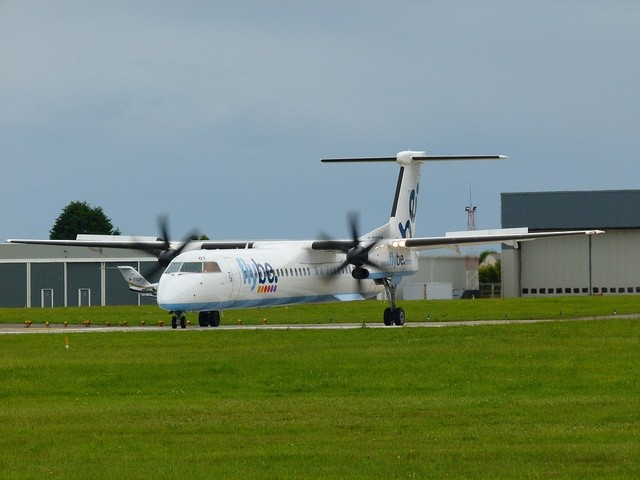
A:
[5,151,547,328]
